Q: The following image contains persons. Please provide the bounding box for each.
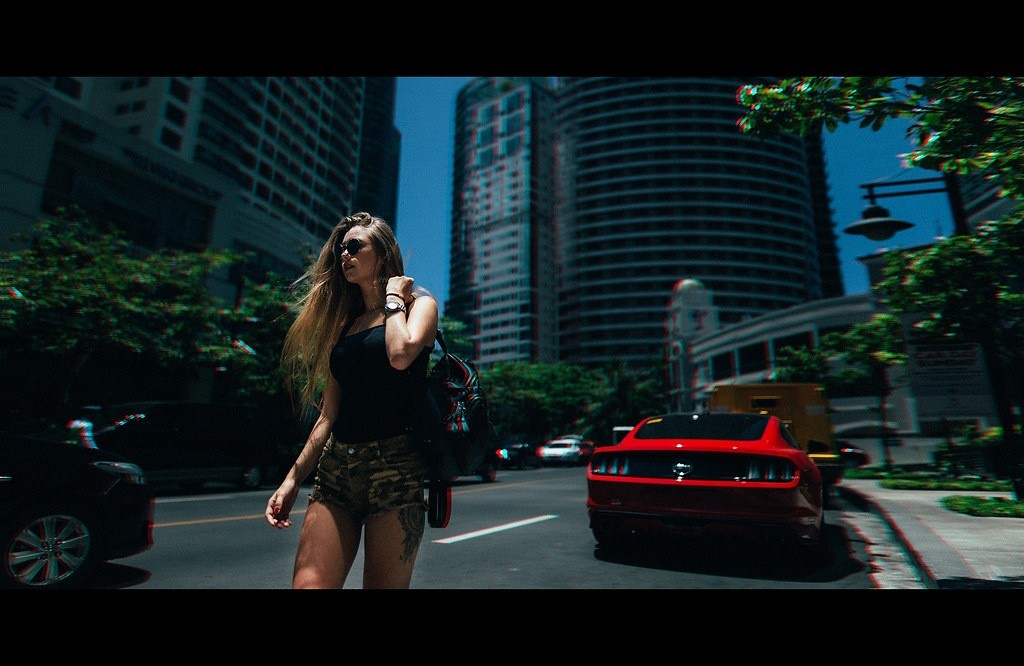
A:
[262,210,440,589]
[62,403,102,451]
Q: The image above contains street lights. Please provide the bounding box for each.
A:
[841,175,987,242]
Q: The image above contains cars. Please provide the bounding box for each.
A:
[495,433,588,468]
[586,411,831,566]
[0,400,154,603]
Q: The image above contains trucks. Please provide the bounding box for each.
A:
[707,383,841,510]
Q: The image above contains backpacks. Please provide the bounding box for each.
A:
[407,331,489,528]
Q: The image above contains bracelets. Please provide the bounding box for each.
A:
[384,292,406,304]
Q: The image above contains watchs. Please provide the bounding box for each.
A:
[383,301,407,317]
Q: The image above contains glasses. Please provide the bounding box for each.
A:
[334,237,374,262]
